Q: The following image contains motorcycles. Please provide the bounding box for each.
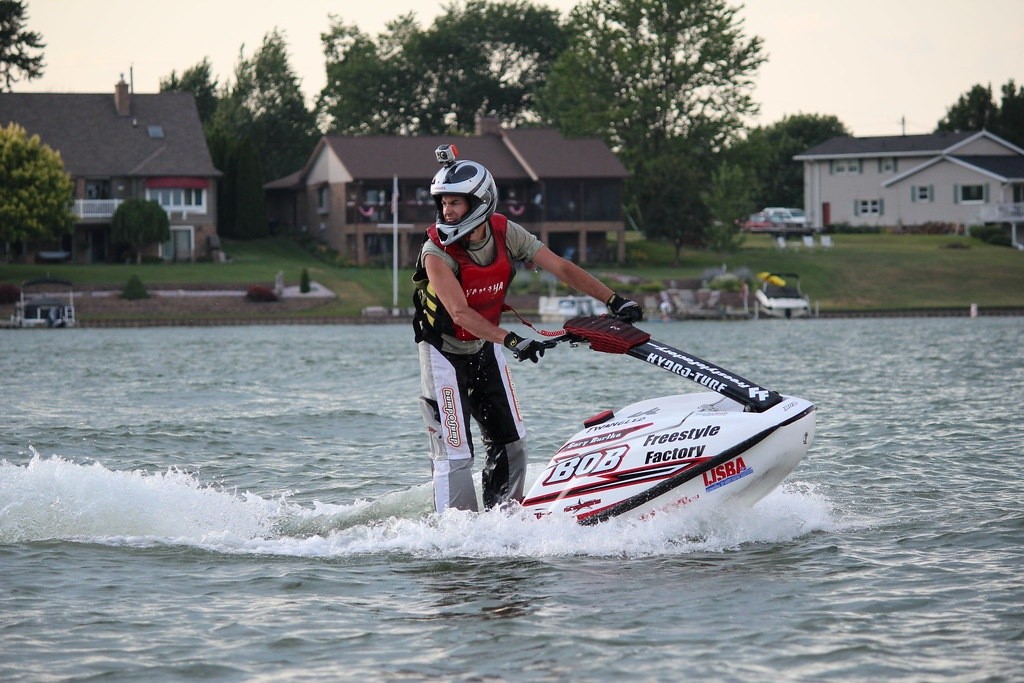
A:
[505,310,818,527]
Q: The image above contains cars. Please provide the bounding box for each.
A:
[740,207,804,232]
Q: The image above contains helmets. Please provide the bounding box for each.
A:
[430,160,497,246]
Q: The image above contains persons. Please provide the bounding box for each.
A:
[409,158,645,512]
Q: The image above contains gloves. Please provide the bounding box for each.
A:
[606,294,642,324]
[504,330,545,363]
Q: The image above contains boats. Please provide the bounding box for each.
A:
[10,278,79,327]
[535,270,815,320]
